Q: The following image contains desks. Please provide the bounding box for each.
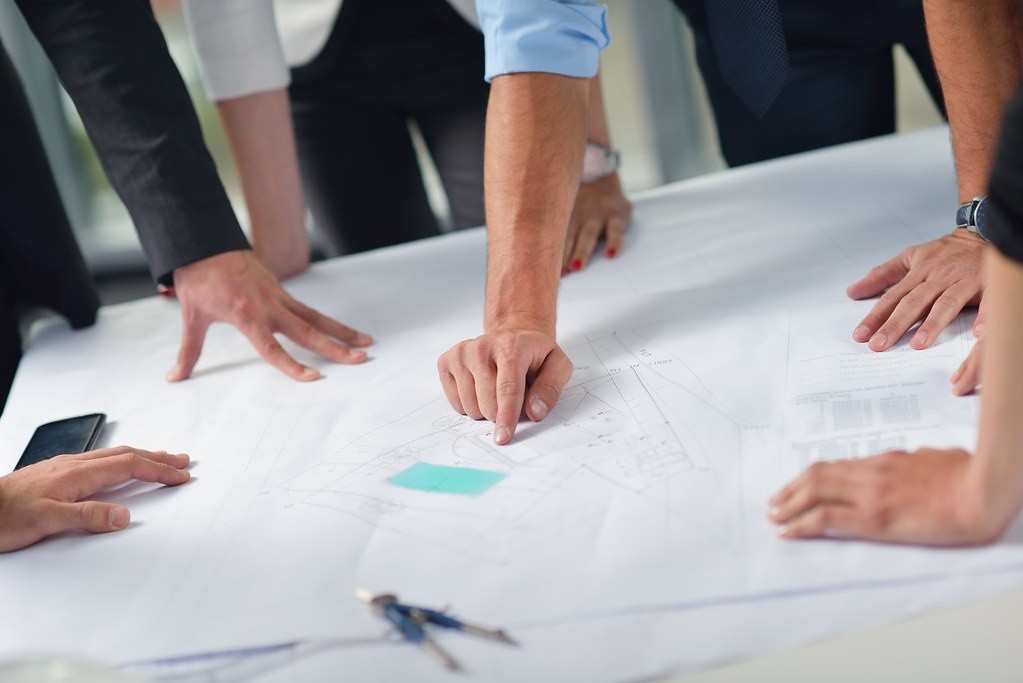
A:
[0,123,1023,683]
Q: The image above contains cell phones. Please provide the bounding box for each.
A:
[12,413,107,471]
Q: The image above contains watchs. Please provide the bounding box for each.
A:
[956,194,990,242]
[580,142,619,182]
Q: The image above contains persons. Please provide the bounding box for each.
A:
[438,0,1023,446]
[0,0,376,552]
[767,83,1022,548]
[180,0,633,277]
